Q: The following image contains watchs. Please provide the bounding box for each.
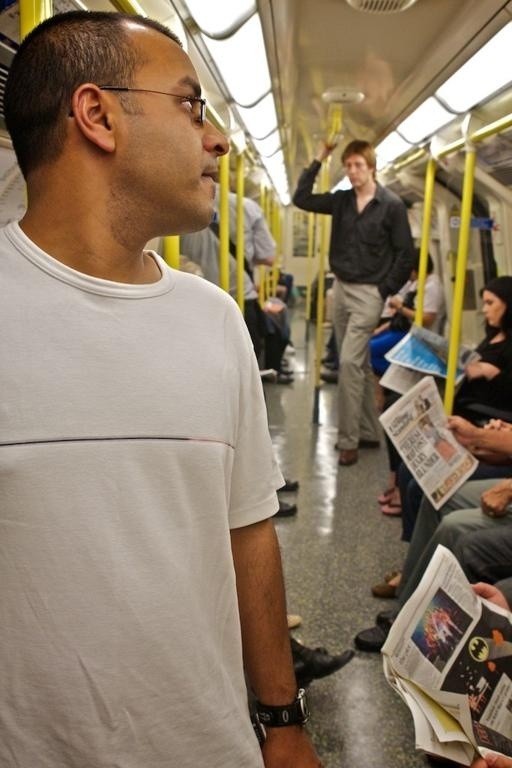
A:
[248,686,312,741]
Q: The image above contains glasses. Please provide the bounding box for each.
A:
[69,86,206,128]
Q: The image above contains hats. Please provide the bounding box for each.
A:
[477,276,512,308]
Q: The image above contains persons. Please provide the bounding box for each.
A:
[290,137,416,465]
[315,245,449,385]
[354,275,511,768]
[1,9,325,767]
[145,180,299,521]
